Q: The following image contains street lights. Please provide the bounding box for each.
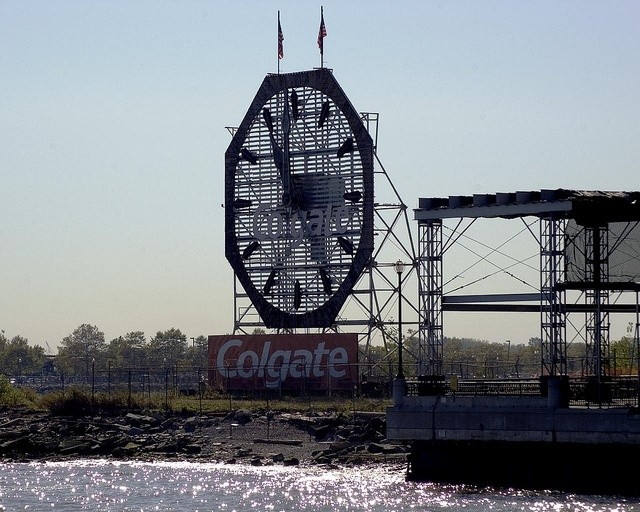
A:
[92,357,95,405]
[19,359,22,375]
[506,340,511,372]
[392,259,406,380]
